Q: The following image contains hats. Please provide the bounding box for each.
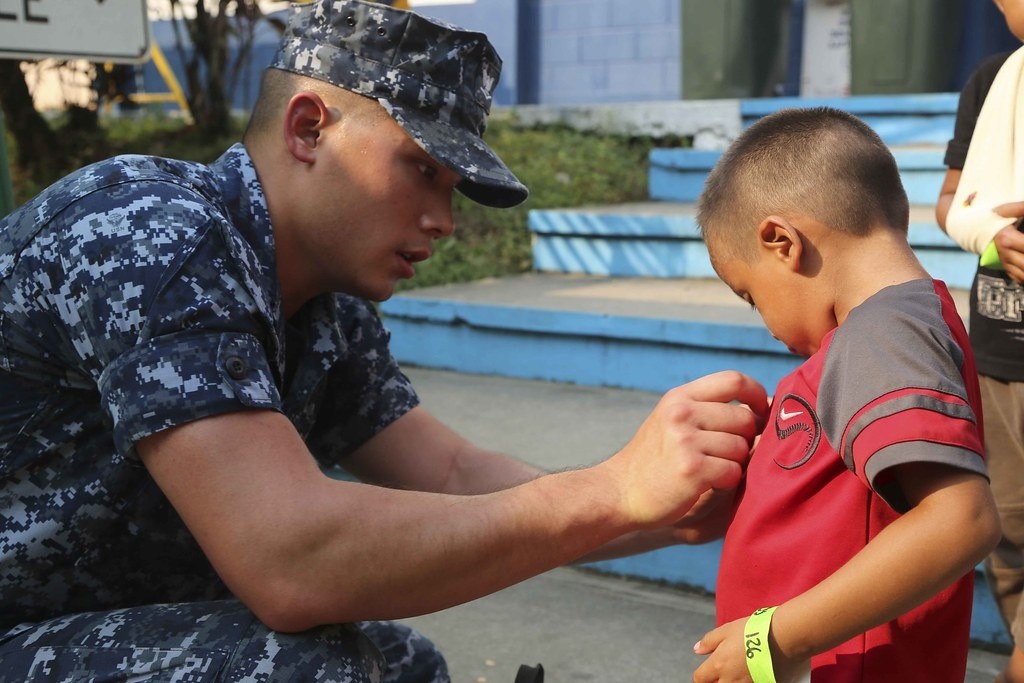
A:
[271,0,529,208]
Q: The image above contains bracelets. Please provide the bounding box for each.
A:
[745,605,812,683]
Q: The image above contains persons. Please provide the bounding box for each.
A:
[936,0,1024,682]
[1,0,772,683]
[695,108,1003,682]
